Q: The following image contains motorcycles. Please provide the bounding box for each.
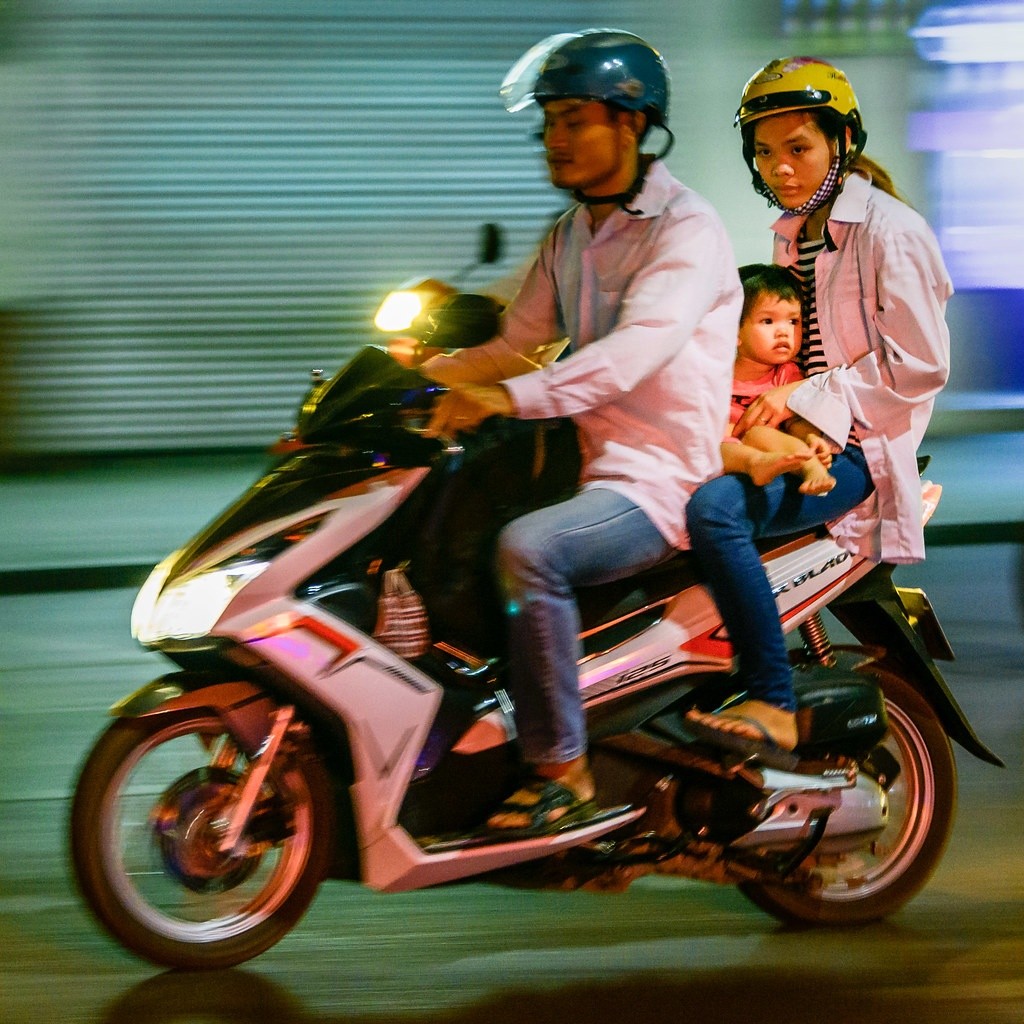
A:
[63,226,1012,965]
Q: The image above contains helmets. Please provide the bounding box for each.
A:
[500,28,668,123]
[733,56,868,163]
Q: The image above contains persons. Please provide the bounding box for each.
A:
[686,56,955,771]
[720,264,837,494]
[413,29,744,838]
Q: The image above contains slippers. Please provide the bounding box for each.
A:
[489,777,596,840]
[683,709,799,771]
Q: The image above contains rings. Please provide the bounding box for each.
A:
[758,417,768,423]
[452,416,468,421]
